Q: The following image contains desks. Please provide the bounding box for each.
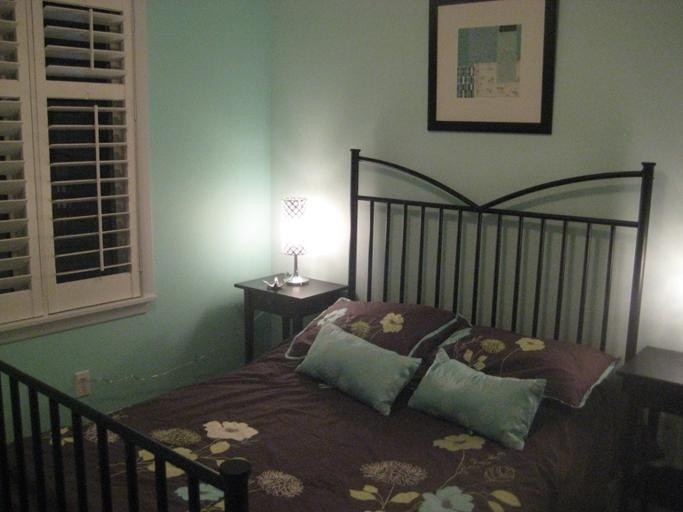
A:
[616,346,683,511]
[234,274,350,367]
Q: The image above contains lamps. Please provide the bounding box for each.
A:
[276,197,313,287]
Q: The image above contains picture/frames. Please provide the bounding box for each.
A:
[426,1,559,134]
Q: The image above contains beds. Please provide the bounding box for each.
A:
[1,149,656,509]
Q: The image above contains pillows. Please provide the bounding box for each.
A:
[293,318,423,416]
[406,348,547,451]
[407,312,618,408]
[285,298,457,359]
